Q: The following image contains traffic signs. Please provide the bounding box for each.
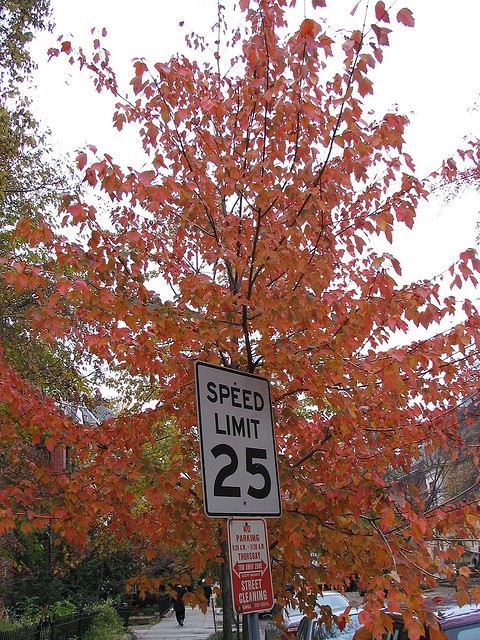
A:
[186,360,281,519]
[223,517,275,618]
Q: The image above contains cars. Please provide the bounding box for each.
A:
[295,606,365,640]
[265,588,355,640]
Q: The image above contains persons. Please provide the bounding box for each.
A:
[172,584,187,627]
[202,582,212,608]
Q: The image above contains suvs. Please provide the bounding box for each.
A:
[377,593,479,639]
